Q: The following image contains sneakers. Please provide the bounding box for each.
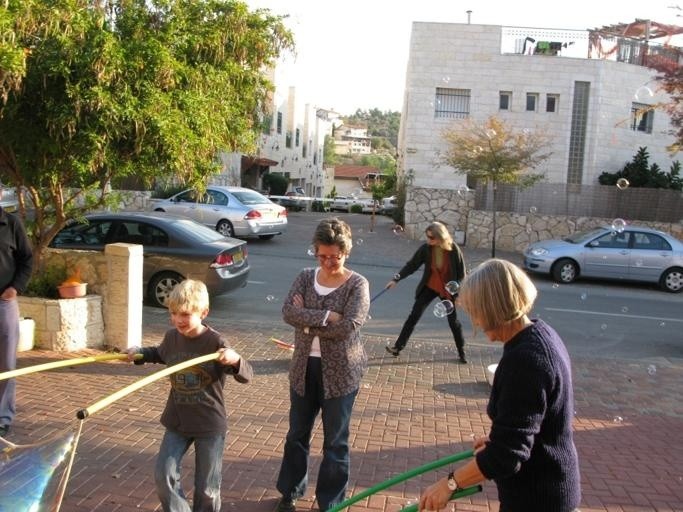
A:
[384,345,401,357]
[458,349,468,365]
[277,496,295,512]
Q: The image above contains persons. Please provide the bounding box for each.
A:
[119,279,254,512]
[0,200,33,437]
[418,259,581,512]
[386,221,467,363]
[274,218,370,512]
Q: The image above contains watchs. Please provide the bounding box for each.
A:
[447,473,463,493]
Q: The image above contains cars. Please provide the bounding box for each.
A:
[46,212,250,309]
[330,196,398,215]
[281,192,307,212]
[524,226,683,293]
[151,185,288,243]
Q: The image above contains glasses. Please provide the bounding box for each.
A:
[427,234,436,241]
[315,250,343,264]
